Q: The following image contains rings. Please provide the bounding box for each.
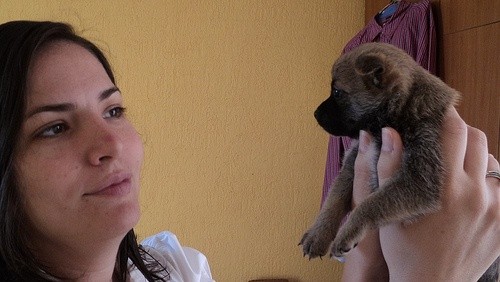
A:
[486,171,500,181]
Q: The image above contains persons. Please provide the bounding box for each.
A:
[0,20,500,282]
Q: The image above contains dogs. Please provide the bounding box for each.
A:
[296,41,500,282]
